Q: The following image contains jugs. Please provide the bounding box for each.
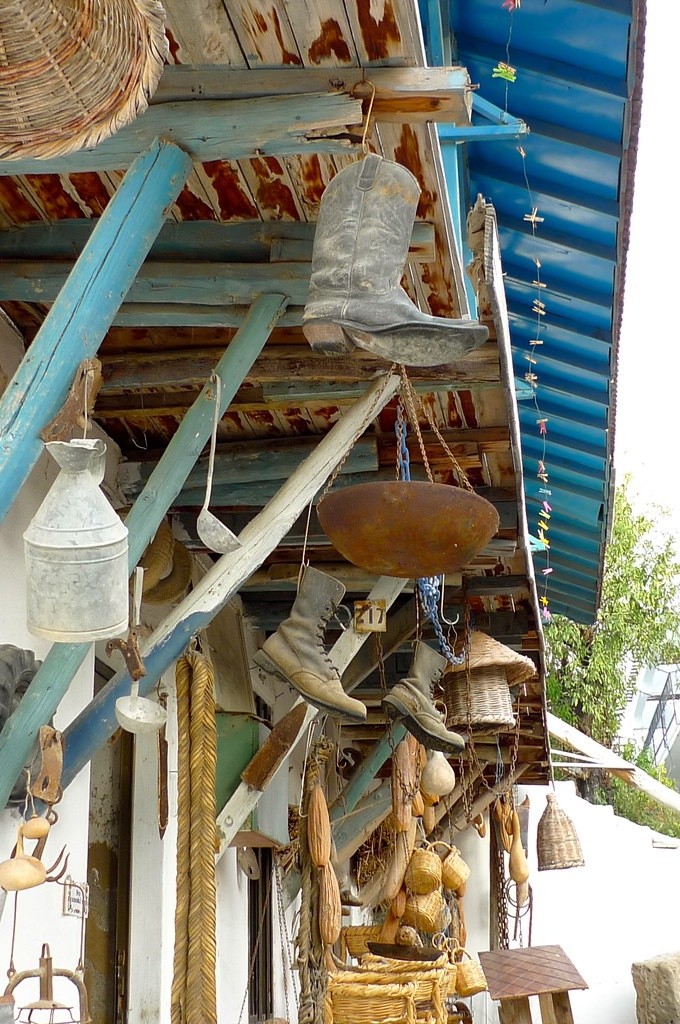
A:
[18,434,134,642]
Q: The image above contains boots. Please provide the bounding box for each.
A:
[302,164,488,367]
[380,641,465,752]
[252,568,366,722]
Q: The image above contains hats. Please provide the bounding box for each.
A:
[434,631,535,701]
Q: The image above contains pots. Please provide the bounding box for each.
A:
[316,479,500,581]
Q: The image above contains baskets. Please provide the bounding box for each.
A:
[439,667,516,737]
[535,793,585,871]
[326,841,486,1024]
